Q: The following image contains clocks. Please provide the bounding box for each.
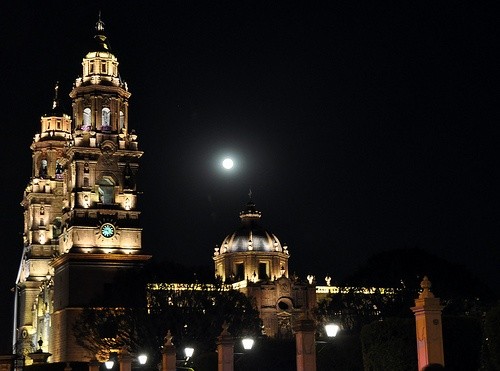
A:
[99,223,117,239]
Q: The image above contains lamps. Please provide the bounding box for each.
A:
[176,346,195,367]
[317,323,342,352]
[234,336,255,363]
[104,358,116,371]
[132,352,150,369]
